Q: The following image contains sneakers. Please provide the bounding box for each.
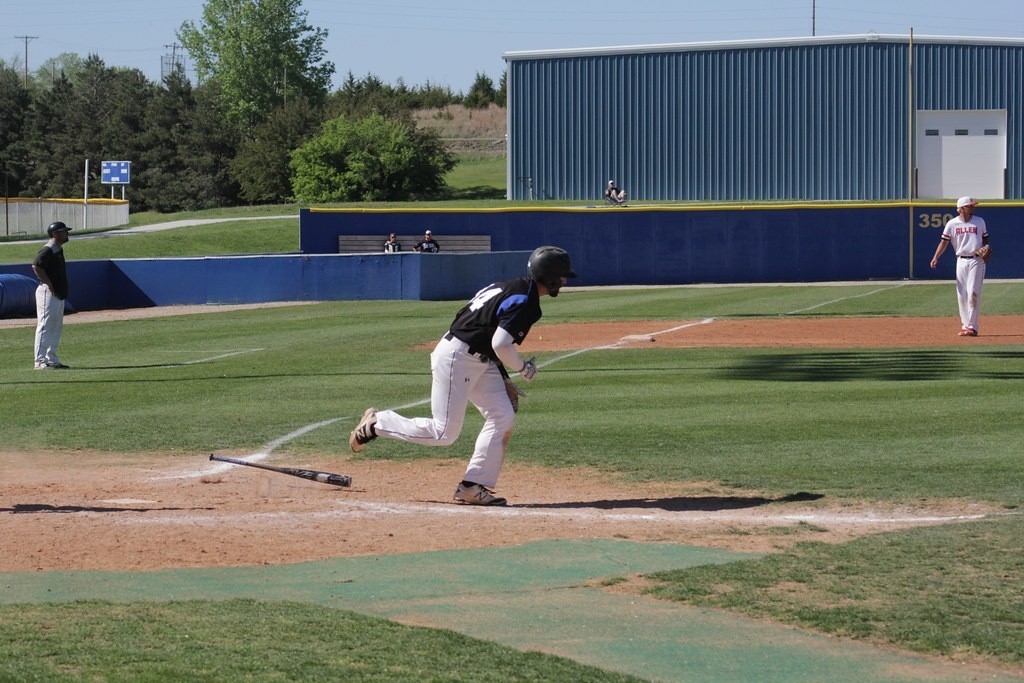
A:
[349,407,378,453]
[454,482,507,505]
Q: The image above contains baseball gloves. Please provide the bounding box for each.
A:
[974,245,992,264]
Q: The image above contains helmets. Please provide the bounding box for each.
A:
[47,222,72,235]
[527,246,578,297]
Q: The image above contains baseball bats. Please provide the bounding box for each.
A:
[208,453,352,487]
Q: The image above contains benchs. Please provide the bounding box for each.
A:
[338,235,493,254]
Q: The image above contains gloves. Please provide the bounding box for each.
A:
[519,357,538,383]
[503,378,526,414]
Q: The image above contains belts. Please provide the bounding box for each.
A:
[444,332,488,362]
[961,254,980,258]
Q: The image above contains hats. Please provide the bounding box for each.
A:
[957,196,979,208]
[425,230,431,235]
[609,181,614,184]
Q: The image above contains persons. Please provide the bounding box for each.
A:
[413,230,440,253]
[32,222,73,369]
[605,180,626,203]
[384,233,402,253]
[350,246,579,506]
[930,196,991,336]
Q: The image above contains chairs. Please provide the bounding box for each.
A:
[604,187,622,207]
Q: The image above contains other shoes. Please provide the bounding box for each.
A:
[50,363,70,369]
[34,362,57,369]
[966,328,977,336]
[958,329,967,336]
